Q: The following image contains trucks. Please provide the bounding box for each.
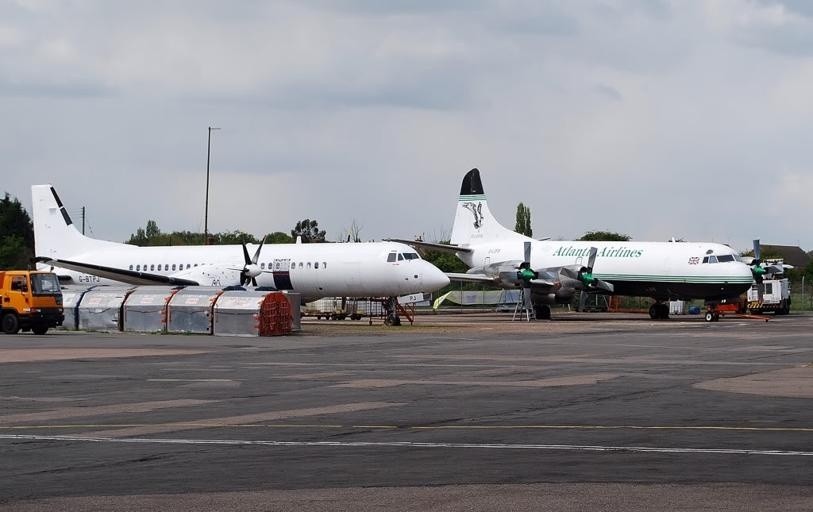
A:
[747,278,791,315]
[0,269,64,334]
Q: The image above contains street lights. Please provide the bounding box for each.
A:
[204,127,220,245]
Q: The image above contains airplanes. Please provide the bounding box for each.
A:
[382,168,753,322]
[31,185,451,326]
